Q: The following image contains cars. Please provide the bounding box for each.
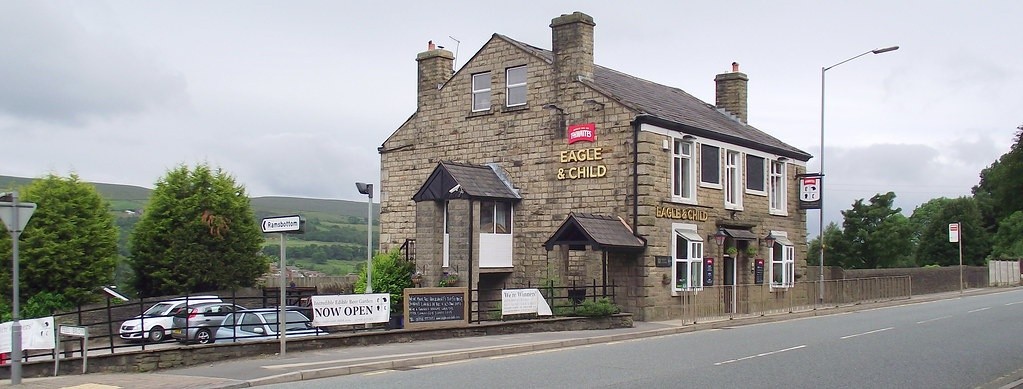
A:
[170,302,247,344]
[215,307,331,345]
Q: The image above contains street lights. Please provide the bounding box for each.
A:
[815,43,901,304]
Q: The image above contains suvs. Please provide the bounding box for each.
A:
[119,295,224,343]
